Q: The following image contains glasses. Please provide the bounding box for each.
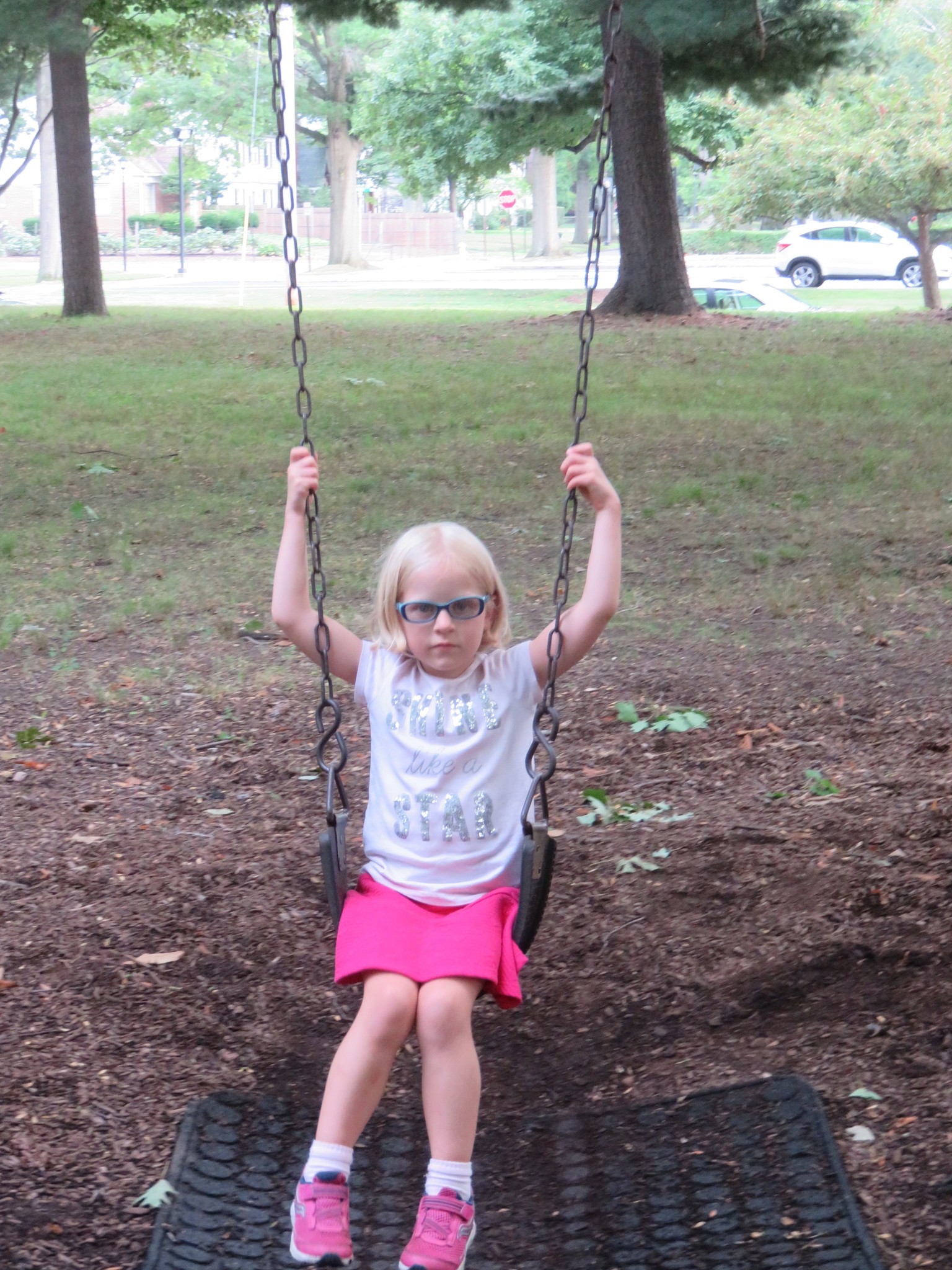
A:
[394,595,490,624]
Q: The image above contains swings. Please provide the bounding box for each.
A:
[262,0,627,987]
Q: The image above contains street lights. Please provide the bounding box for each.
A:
[173,126,193,273]
[693,172,698,229]
[117,157,128,272]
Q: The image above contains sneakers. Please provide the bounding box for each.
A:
[398,1186,476,1270]
[290,1162,354,1265]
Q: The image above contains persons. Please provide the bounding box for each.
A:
[272,440,622,1270]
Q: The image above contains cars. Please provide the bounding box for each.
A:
[689,280,813,313]
[774,220,952,288]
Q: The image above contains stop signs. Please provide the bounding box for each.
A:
[498,190,516,208]
